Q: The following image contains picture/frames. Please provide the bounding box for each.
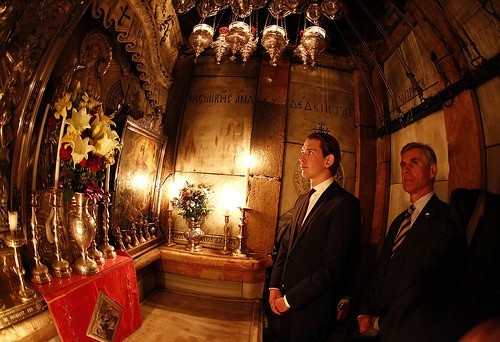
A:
[108,114,168,243]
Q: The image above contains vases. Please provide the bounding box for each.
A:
[183,214,206,252]
[66,191,99,275]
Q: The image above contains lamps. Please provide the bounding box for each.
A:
[175,0,337,71]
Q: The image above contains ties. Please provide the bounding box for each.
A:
[296,188,316,236]
[391,205,415,252]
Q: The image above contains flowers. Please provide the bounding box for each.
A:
[50,79,125,193]
[170,180,215,220]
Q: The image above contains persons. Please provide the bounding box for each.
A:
[356,143,468,342]
[268,133,362,342]
[68,44,102,108]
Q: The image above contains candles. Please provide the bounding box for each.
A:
[7,210,18,232]
[225,207,230,216]
[168,200,173,210]
